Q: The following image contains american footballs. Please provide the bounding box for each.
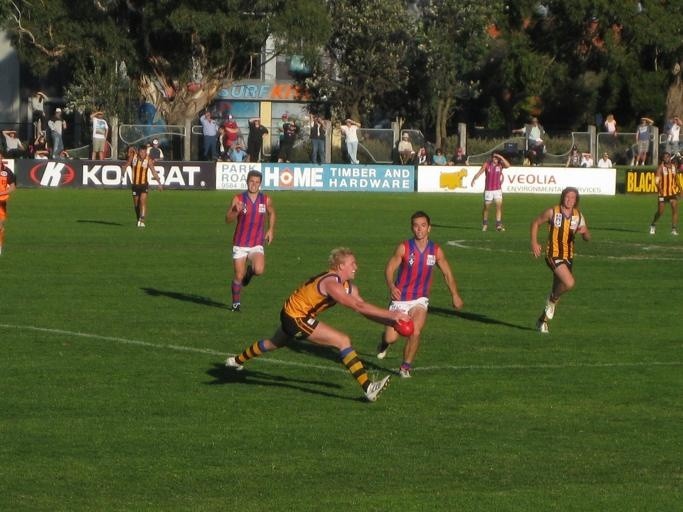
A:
[395,319,414,337]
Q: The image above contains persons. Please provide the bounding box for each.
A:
[276,112,300,163]
[512,117,546,167]
[525,139,546,167]
[448,146,468,165]
[340,119,362,164]
[530,186,590,333]
[635,117,654,167]
[225,247,412,402]
[565,144,582,168]
[28,88,50,142]
[199,111,220,162]
[432,148,447,166]
[376,209,463,380]
[245,118,269,162]
[32,135,48,151]
[597,152,613,168]
[122,144,163,227]
[310,116,327,163]
[603,114,619,138]
[0,150,17,256]
[33,150,50,160]
[397,132,413,165]
[146,137,164,162]
[3,129,26,160]
[54,150,70,159]
[225,168,275,313]
[469,152,511,233]
[648,151,683,237]
[666,117,683,156]
[90,110,109,161]
[227,146,248,162]
[417,146,428,165]
[580,152,594,168]
[48,107,67,159]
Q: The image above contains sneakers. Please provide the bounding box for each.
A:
[375,343,389,359]
[240,264,254,287]
[400,365,412,378]
[536,320,550,336]
[230,302,243,315]
[649,225,656,235]
[135,221,146,229]
[224,356,244,373]
[671,227,679,236]
[495,226,506,232]
[364,369,389,401]
[481,224,487,232]
[544,296,557,321]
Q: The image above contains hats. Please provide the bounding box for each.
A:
[345,118,352,123]
[56,108,62,113]
[402,132,409,138]
[281,114,288,118]
[532,117,538,121]
[153,139,158,145]
